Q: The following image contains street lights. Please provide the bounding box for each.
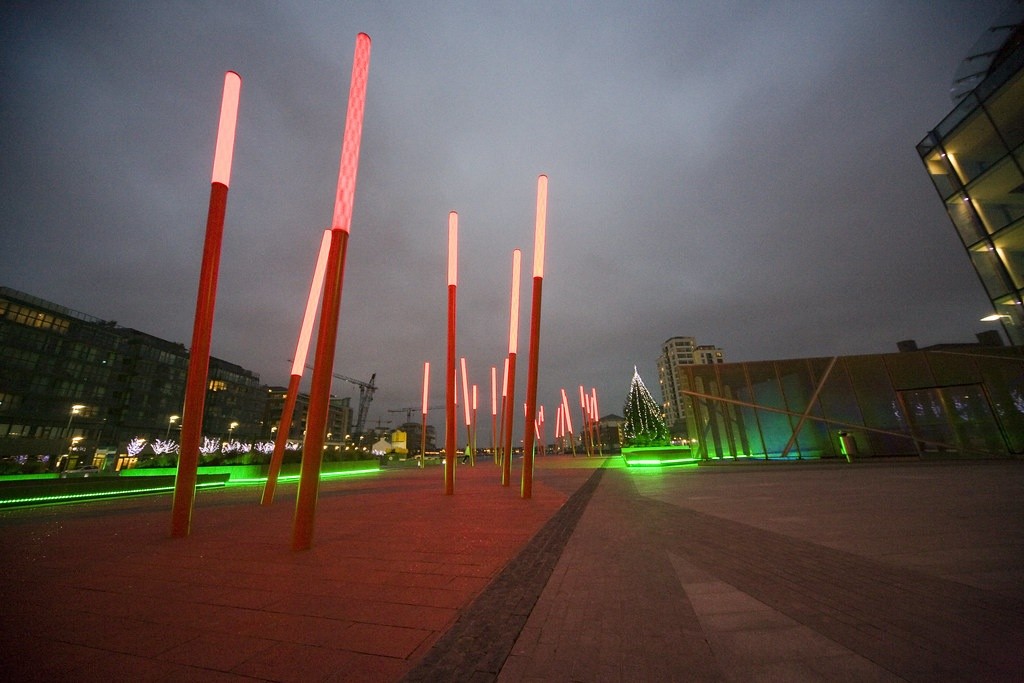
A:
[228,421,238,442]
[59,402,85,455]
[269,426,279,442]
[164,414,180,443]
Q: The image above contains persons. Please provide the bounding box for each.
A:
[462,444,470,465]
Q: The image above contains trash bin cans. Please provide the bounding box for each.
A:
[835,429,861,464]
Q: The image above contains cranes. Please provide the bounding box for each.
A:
[288,358,379,442]
[387,405,460,423]
[367,418,394,435]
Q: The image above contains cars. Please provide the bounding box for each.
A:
[59,464,101,478]
